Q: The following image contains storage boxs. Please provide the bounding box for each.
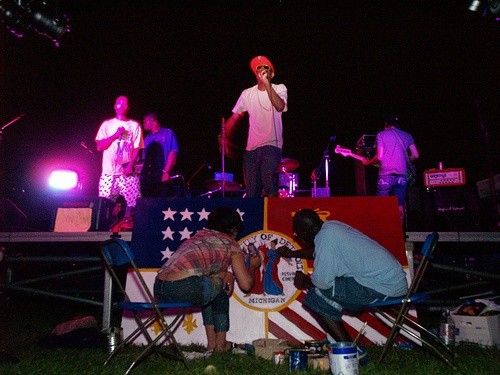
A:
[447,313,500,346]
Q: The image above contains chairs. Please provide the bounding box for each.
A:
[99,239,192,375]
[366,232,460,371]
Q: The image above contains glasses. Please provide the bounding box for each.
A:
[256,65,270,70]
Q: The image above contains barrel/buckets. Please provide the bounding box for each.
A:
[287,340,330,371]
[100,326,124,354]
[327,340,359,375]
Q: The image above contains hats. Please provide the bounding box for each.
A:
[249,55,275,79]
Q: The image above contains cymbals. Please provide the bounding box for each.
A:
[278,158,300,172]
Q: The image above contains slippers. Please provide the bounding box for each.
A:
[228,342,239,353]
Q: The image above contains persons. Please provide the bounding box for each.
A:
[274,209,409,363]
[142,112,179,198]
[94,95,144,221]
[154,207,261,355]
[218,56,288,197]
[362,115,419,241]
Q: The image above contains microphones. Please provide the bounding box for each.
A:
[117,134,122,144]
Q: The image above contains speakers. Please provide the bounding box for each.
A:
[355,148,379,196]
[47,195,116,232]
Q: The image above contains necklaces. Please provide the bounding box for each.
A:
[257,91,271,111]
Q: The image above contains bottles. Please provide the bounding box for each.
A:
[439,309,455,347]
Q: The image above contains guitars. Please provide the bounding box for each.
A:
[334,144,379,167]
